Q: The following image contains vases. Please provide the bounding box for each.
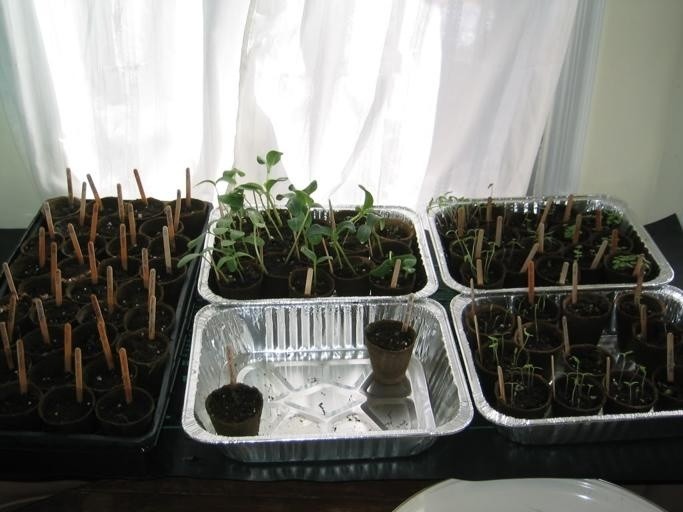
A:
[363,319,417,386]
[205,382,261,436]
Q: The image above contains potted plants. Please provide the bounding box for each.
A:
[176,150,418,298]
[1,197,208,435]
[427,183,676,418]
